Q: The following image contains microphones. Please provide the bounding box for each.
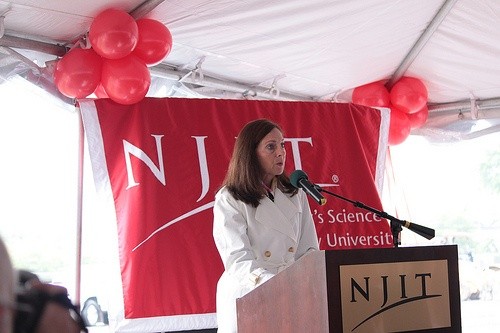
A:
[290,170,327,206]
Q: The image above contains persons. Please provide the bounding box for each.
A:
[0,239,88,333]
[212,118,320,333]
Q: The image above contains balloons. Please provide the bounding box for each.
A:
[352,76,429,145]
[53,6,172,104]
